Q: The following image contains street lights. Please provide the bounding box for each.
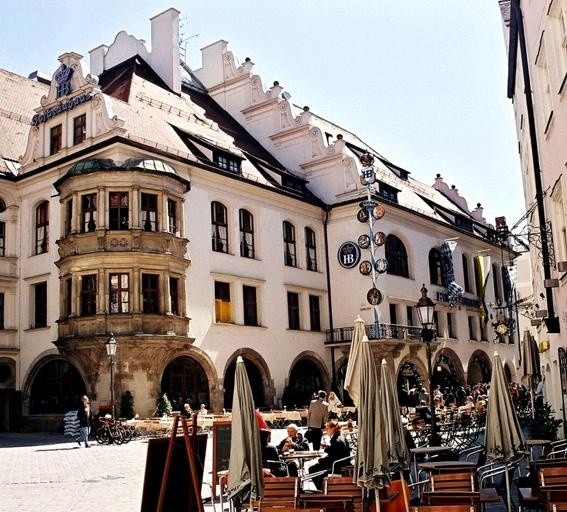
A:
[414,283,441,446]
[105,333,118,421]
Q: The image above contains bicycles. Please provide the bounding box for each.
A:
[95,418,132,445]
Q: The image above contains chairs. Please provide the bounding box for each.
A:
[218,407,567,511]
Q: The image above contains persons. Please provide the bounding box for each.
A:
[75,394,94,448]
[260,373,543,490]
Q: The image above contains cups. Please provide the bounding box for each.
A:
[289,449,294,457]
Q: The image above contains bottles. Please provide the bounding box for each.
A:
[348,418,352,432]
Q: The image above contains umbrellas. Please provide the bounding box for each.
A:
[484,350,531,511]
[228,355,265,512]
[344,314,411,511]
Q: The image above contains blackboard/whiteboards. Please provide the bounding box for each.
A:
[213,421,232,485]
